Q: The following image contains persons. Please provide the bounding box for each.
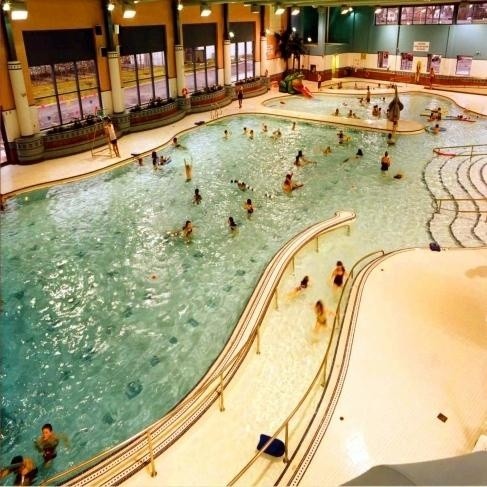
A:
[0,457,39,486]
[106,119,120,156]
[315,301,330,335]
[182,189,253,232]
[35,424,68,467]
[135,66,475,192]
[330,261,350,298]
[52,115,101,133]
[295,276,309,294]
[0,193,7,212]
